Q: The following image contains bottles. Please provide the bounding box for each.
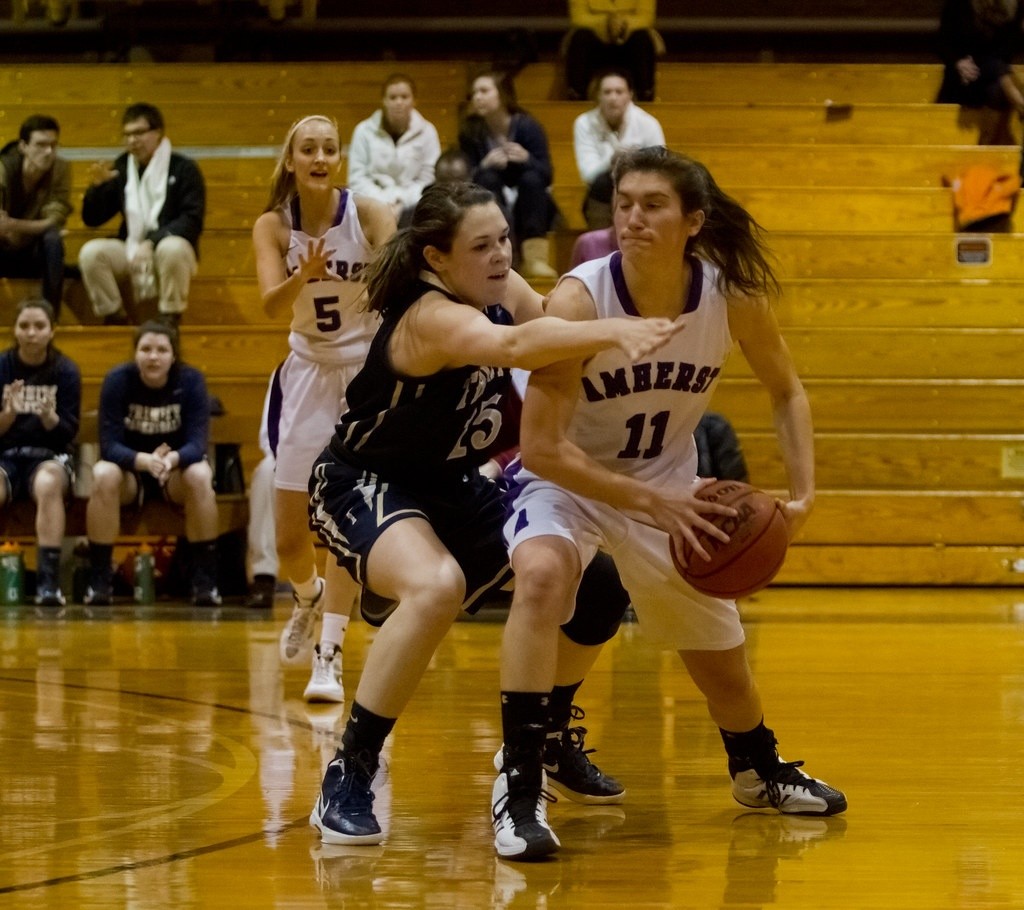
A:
[133,540,154,603]
[0,541,22,608]
[131,239,156,302]
[71,543,90,605]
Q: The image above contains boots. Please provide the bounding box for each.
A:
[520,236,558,285]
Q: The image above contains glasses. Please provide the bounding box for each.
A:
[121,128,156,141]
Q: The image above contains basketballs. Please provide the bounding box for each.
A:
[667,479,789,601]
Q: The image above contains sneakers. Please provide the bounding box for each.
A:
[85,607,113,625]
[726,749,848,816]
[311,842,385,910]
[490,718,626,804]
[302,642,345,704]
[193,582,221,607]
[247,581,275,610]
[250,610,275,631]
[492,854,570,910]
[548,804,626,857]
[309,759,383,847]
[32,580,68,607]
[279,577,325,661]
[193,608,223,627]
[36,608,66,626]
[491,763,562,861]
[84,586,115,605]
[731,809,850,860]
[302,707,345,749]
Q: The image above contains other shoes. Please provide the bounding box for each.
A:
[157,314,179,326]
[107,307,129,326]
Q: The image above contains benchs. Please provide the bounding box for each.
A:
[0,64,1024,588]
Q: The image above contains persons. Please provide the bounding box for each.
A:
[0,114,72,327]
[490,144,847,857]
[307,181,687,845]
[575,224,618,266]
[0,299,82,606]
[83,318,223,605]
[252,116,398,702]
[345,75,442,225]
[78,103,205,327]
[573,66,667,187]
[936,0,1024,118]
[558,1,667,103]
[458,69,570,282]
